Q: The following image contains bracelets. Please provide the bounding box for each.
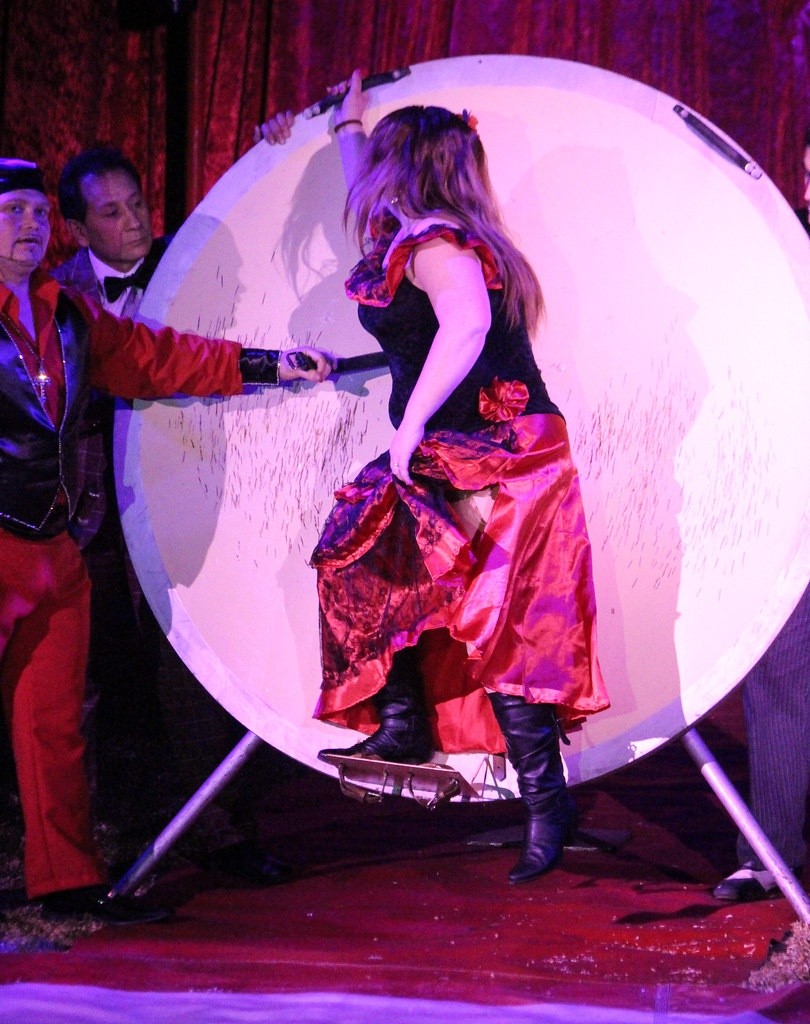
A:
[332,119,363,133]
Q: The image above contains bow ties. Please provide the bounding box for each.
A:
[104,264,154,303]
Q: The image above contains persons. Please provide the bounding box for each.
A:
[315,71,611,886]
[716,586,809,900]
[0,158,338,924]
[51,110,298,884]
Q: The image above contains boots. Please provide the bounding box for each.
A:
[486,692,569,883]
[318,645,432,766]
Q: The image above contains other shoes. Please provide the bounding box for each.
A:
[42,884,176,926]
[713,860,803,900]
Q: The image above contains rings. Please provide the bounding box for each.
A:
[392,463,400,469]
